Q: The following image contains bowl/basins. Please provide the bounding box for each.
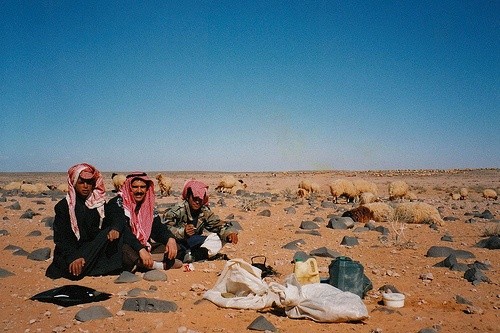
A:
[382,293,405,307]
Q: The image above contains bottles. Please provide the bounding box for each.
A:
[327,257,374,301]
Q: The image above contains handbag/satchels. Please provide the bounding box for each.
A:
[327,256,372,299]
[250,255,268,277]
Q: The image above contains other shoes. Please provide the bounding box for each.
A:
[183,254,193,263]
[130,264,143,273]
[163,259,182,269]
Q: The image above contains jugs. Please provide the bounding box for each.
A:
[294,257,321,284]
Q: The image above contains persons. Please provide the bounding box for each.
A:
[164,181,238,263]
[107,171,183,273]
[45,162,126,281]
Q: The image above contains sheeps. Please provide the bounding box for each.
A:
[481,189,498,200]
[238,180,247,189]
[342,202,395,222]
[367,168,473,177]
[311,169,366,177]
[3,180,69,196]
[111,173,127,193]
[449,188,468,201]
[271,171,305,178]
[296,180,319,198]
[155,173,173,197]
[329,181,380,204]
[388,180,407,201]
[395,202,445,227]
[215,181,234,194]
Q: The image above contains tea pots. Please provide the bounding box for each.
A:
[250,255,272,279]
